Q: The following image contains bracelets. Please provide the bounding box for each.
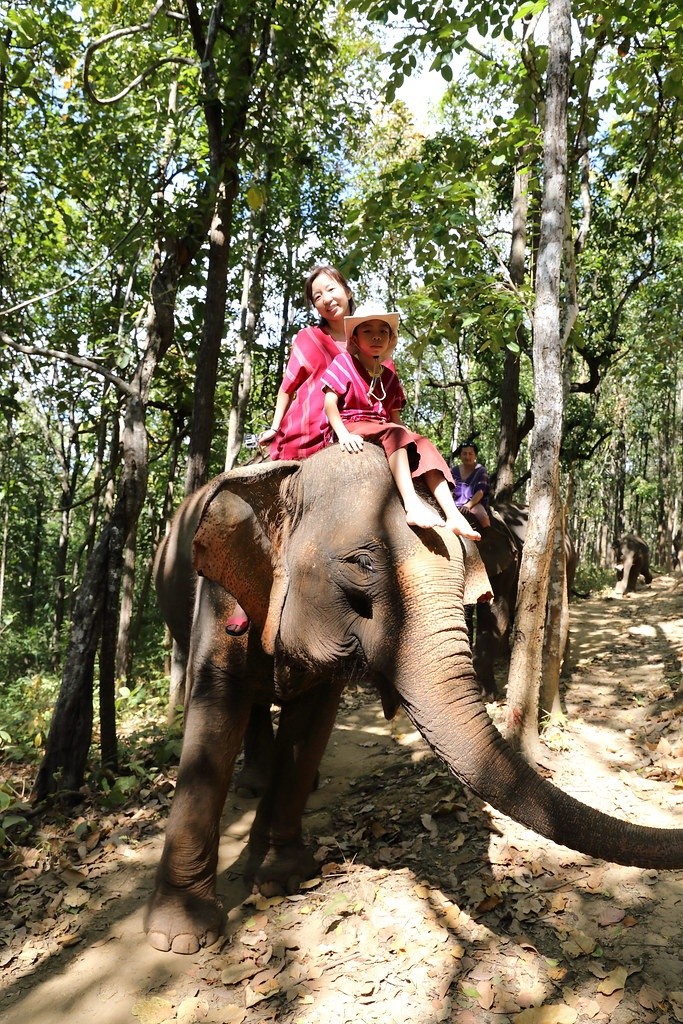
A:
[271,428,277,433]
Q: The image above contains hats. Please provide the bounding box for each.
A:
[344,298,399,363]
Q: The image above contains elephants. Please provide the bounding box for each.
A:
[141,440,683,955]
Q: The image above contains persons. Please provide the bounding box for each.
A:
[258,266,357,462]
[450,441,491,528]
[320,302,481,540]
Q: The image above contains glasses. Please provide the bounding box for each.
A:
[462,442,476,449]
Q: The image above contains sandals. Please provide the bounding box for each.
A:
[224,615,249,636]
